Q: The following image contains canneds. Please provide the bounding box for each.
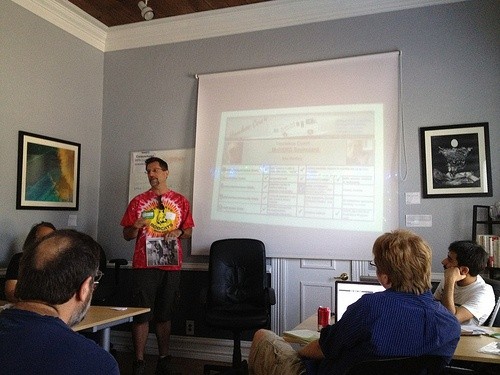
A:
[317,306,331,331]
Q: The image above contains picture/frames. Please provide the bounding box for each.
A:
[419,122,493,198]
[16,131,81,211]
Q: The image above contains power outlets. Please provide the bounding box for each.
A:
[186,320,194,335]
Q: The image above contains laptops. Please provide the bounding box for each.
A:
[335,281,386,323]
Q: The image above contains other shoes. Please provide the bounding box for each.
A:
[156,355,184,375]
[132,359,146,375]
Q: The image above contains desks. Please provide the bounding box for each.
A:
[283,307,500,375]
[0,301,151,353]
[359,271,447,286]
[0,260,273,277]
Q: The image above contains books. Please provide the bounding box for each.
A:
[283,330,320,344]
[476,234,500,267]
[461,325,500,355]
[479,269,500,286]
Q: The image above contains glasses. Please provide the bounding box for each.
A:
[94,271,104,282]
[370,260,377,266]
[145,168,164,174]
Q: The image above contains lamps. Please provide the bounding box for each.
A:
[137,0,154,21]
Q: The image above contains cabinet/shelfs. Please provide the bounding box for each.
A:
[472,205,500,272]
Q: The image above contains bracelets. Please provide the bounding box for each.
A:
[176,228,184,238]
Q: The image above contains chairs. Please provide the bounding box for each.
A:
[482,279,500,327]
[91,259,128,351]
[199,239,276,375]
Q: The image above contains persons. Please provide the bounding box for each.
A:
[248,228,461,375]
[433,240,496,327]
[0,229,121,375]
[5,221,56,303]
[120,156,196,375]
